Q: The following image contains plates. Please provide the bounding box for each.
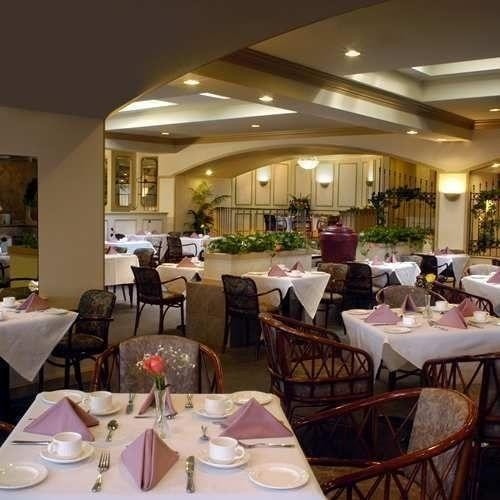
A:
[36,389,86,404]
[195,405,238,419]
[40,442,94,464]
[78,397,123,415]
[44,308,69,315]
[248,271,325,278]
[348,306,496,334]
[196,446,249,469]
[0,461,49,489]
[247,462,309,490]
[231,390,272,405]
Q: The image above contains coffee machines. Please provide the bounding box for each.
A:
[82,390,113,412]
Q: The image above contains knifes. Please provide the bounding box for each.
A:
[184,455,195,494]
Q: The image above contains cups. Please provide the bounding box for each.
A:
[435,301,449,309]
[48,431,83,459]
[204,393,233,414]
[208,436,245,465]
[0,297,16,320]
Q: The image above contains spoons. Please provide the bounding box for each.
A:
[105,419,118,443]
[199,424,210,441]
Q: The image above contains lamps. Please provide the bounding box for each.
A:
[315,167,334,187]
[257,166,272,186]
[366,171,374,187]
[298,154,318,169]
[437,173,468,202]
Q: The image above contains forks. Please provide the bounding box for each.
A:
[236,440,295,449]
[185,394,193,408]
[91,450,111,493]
[126,393,136,414]
